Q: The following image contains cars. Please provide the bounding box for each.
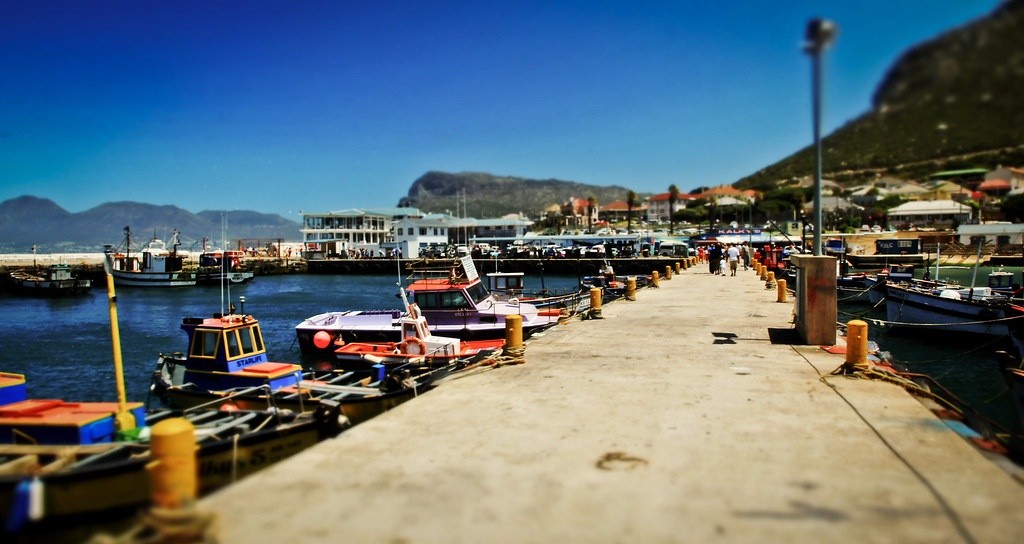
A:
[784,245,811,252]
[423,243,696,258]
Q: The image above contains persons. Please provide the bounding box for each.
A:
[274,246,303,259]
[696,241,751,277]
[348,246,402,261]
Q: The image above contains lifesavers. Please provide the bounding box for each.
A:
[401,337,426,355]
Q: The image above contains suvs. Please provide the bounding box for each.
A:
[824,238,846,254]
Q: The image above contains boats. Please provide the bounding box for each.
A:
[0,228,652,544]
[782,252,1023,430]
